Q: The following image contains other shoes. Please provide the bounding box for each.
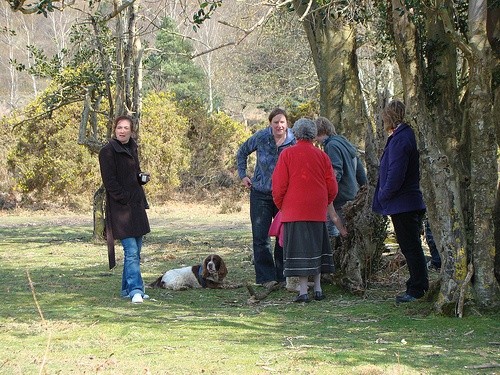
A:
[143,294,150,298]
[263,280,278,288]
[312,289,323,300]
[132,293,143,302]
[293,294,311,302]
[398,294,414,302]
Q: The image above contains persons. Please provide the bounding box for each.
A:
[236,108,297,287]
[372,100,441,301]
[315,117,372,204]
[98,116,150,303]
[271,118,347,303]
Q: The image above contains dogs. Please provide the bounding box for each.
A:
[144,254,244,289]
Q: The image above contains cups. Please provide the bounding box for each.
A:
[141,174,151,182]
